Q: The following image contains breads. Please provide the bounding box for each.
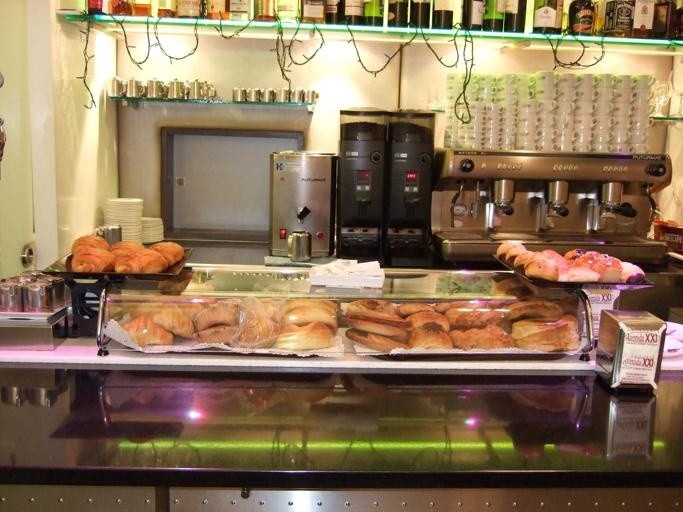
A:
[72,234,185,276]
[496,239,645,284]
[103,292,581,350]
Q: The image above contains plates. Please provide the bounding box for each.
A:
[138,216,164,245]
[104,196,144,249]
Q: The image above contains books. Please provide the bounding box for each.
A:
[309,259,385,290]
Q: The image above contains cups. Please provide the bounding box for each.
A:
[445,70,657,156]
[286,231,314,262]
[106,73,217,103]
[0,268,64,313]
[231,88,317,103]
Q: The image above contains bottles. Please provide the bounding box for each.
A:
[58,0,680,43]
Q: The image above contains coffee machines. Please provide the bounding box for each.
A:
[336,107,388,262]
[433,149,674,270]
[388,110,437,268]
